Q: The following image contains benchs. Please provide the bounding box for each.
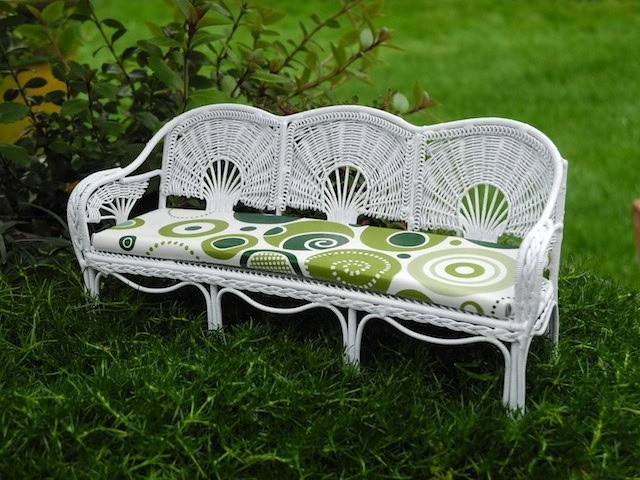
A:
[66,102,574,418]
